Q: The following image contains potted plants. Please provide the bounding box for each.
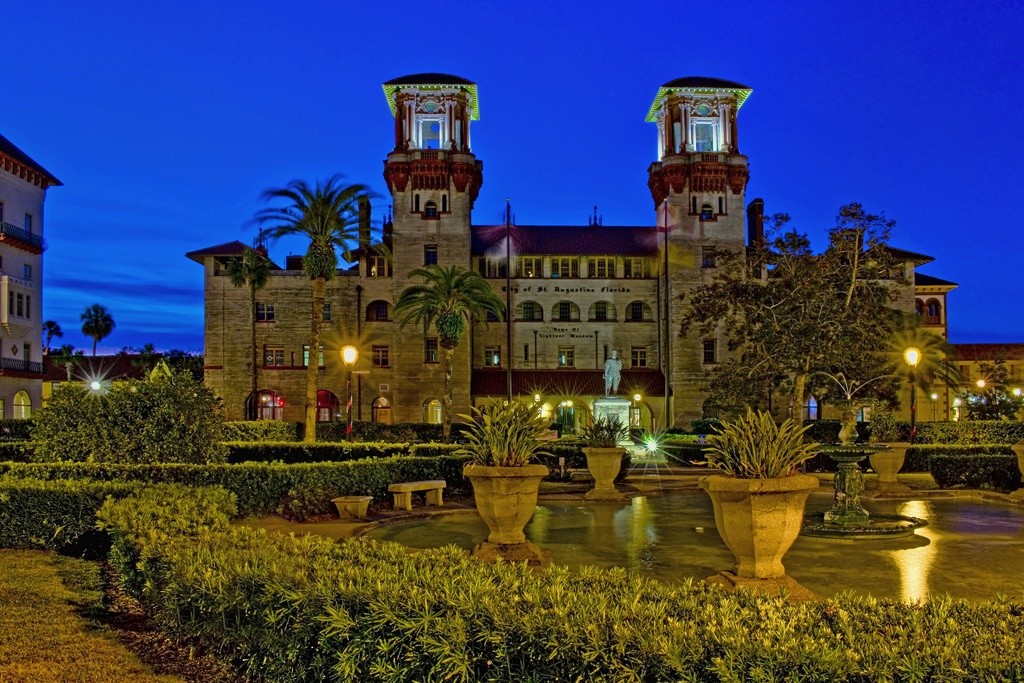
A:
[695,407,824,578]
[576,413,629,501]
[456,394,556,544]
[1011,439,1024,482]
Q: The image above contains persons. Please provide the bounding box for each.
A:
[605,350,622,398]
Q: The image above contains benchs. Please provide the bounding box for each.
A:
[389,480,447,510]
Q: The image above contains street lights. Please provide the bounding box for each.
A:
[931,394,937,422]
[339,346,360,441]
[903,348,922,442]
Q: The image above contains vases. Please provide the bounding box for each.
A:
[332,495,372,519]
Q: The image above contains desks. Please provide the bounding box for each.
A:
[869,402,911,484]
